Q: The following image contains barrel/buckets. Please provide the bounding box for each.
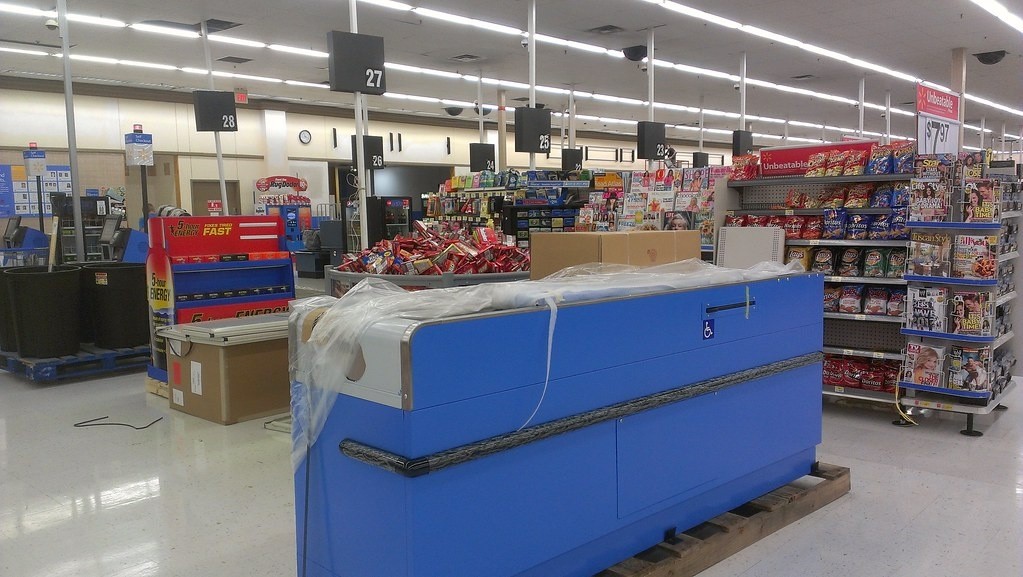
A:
[0,263,148,358]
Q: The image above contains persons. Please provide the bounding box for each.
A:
[915,159,924,174]
[606,191,618,212]
[961,357,983,391]
[974,153,984,178]
[963,152,974,178]
[965,182,980,223]
[690,170,703,192]
[685,197,700,213]
[905,348,939,382]
[978,180,996,220]
[138,203,160,234]
[669,214,687,230]
[953,294,966,335]
[921,186,938,221]
[911,186,922,222]
[663,170,674,190]
[639,171,649,187]
[963,293,988,333]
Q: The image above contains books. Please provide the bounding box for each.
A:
[910,150,1001,221]
[906,285,950,337]
[904,283,998,341]
[902,341,990,392]
[574,165,732,244]
[906,232,998,280]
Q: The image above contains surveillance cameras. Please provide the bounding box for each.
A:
[474,100,478,105]
[998,137,1001,139]
[881,113,885,117]
[976,133,981,135]
[44,20,59,30]
[521,40,528,47]
[734,83,740,90]
[641,65,647,72]
[565,109,569,114]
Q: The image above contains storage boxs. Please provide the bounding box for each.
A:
[530,230,702,280]
[717,227,785,269]
[156,312,290,426]
[336,220,531,276]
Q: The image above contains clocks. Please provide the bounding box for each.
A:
[299,130,311,144]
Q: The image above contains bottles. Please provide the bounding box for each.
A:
[386,212,406,239]
[259,194,311,207]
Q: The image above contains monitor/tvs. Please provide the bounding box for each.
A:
[3,216,22,239]
[99,214,122,244]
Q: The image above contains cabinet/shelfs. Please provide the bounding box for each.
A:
[420,181,592,249]
[714,160,1023,436]
